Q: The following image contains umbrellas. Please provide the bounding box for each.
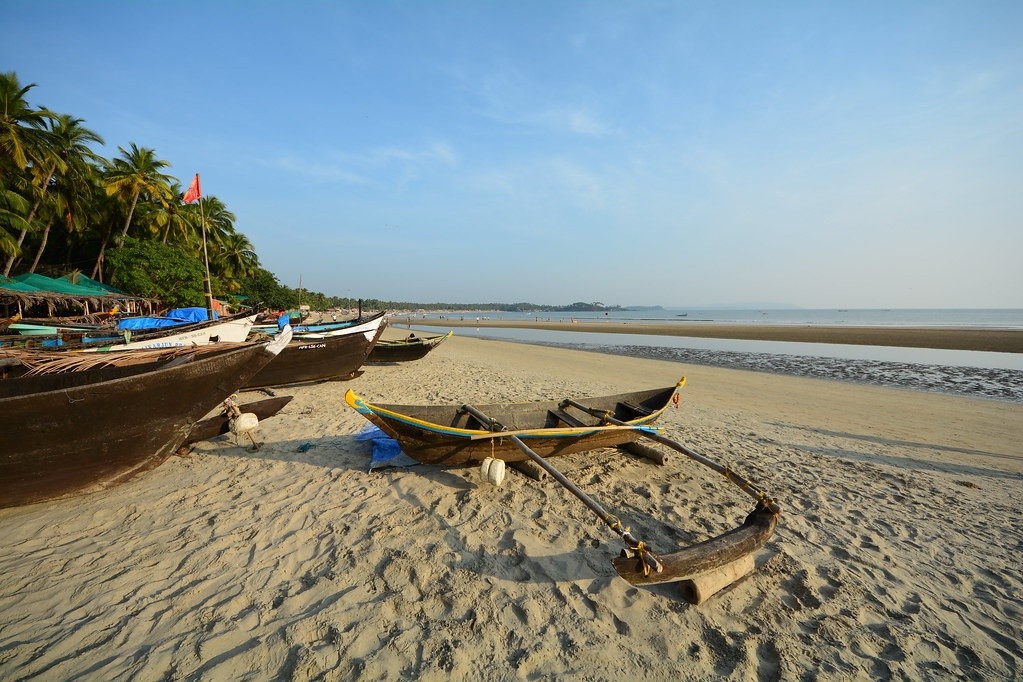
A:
[0,273,146,320]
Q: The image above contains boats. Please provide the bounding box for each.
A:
[345,375,782,587]
[0,298,455,396]
[0,310,294,510]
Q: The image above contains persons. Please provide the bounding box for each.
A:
[476,316,479,323]
[333,314,336,321]
[481,316,490,320]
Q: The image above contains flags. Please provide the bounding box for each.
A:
[184,175,201,204]
